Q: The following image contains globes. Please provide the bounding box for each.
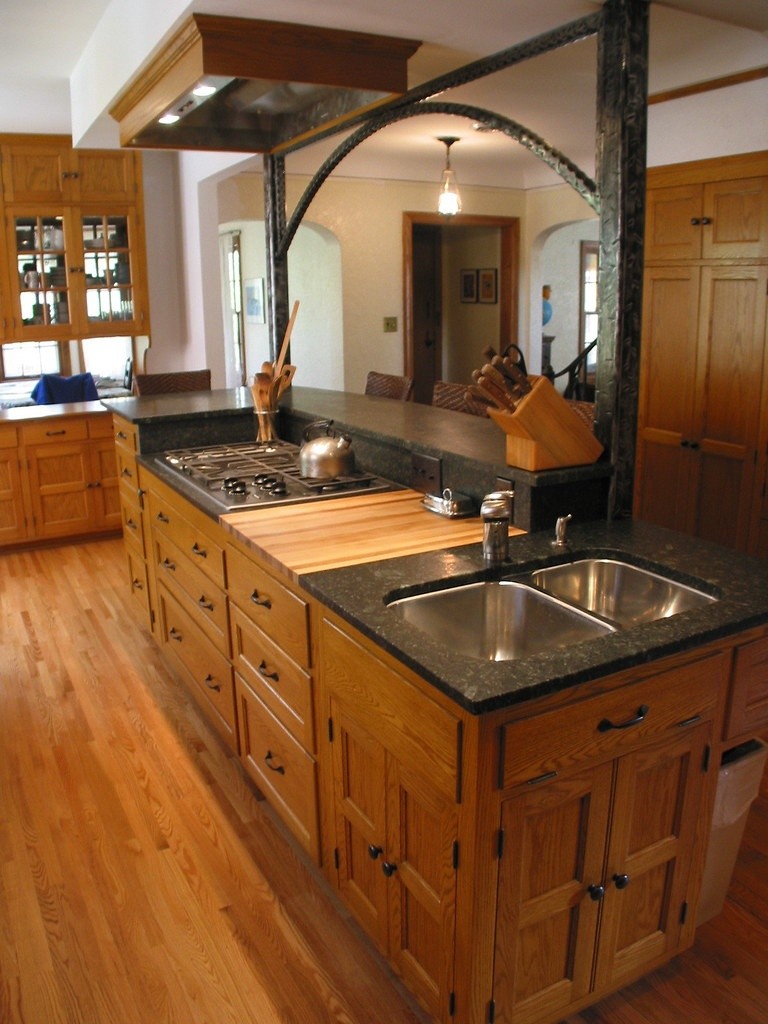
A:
[542,301,553,328]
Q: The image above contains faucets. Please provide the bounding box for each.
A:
[480,489,516,562]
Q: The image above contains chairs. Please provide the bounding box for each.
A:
[132,369,212,395]
[363,372,415,399]
[432,381,492,418]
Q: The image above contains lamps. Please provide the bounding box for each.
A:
[437,136,462,215]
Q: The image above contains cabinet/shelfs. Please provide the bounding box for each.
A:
[631,149,767,557]
[113,411,768,1024]
[0,413,120,546]
[0,133,150,347]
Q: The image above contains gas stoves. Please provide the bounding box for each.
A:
[153,438,408,520]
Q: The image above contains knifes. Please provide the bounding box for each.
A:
[467,345,532,415]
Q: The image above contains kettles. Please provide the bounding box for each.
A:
[300,420,355,479]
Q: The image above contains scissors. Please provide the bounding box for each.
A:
[499,343,529,384]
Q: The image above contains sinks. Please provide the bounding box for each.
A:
[385,576,619,662]
[530,557,719,629]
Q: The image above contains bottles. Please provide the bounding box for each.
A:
[34,226,54,250]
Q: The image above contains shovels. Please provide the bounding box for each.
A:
[251,361,297,442]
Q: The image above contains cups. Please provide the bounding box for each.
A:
[38,273,51,288]
[104,270,113,284]
[101,312,120,321]
[121,301,133,320]
[24,271,38,288]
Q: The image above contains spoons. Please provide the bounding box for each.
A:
[261,362,273,377]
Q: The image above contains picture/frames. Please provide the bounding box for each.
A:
[460,269,477,302]
[476,268,497,303]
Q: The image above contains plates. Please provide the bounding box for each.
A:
[84,240,113,249]
[50,268,65,286]
